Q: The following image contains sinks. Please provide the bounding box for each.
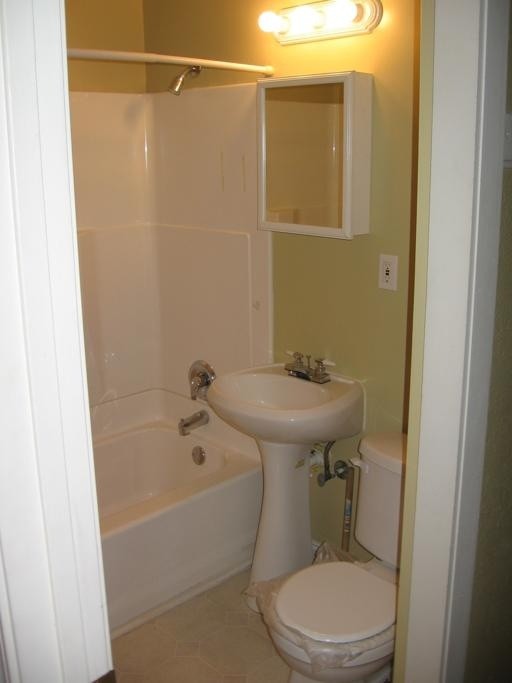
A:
[208,362,363,445]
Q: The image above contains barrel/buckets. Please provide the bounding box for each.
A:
[178,409,207,436]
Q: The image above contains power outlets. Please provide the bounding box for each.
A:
[378,253,398,291]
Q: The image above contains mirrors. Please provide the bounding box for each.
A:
[257,71,373,241]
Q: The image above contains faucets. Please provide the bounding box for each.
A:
[283,362,309,375]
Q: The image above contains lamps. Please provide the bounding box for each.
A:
[257,0,383,47]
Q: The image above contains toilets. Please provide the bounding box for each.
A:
[265,432,404,683]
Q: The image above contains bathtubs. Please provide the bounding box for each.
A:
[89,389,264,638]
